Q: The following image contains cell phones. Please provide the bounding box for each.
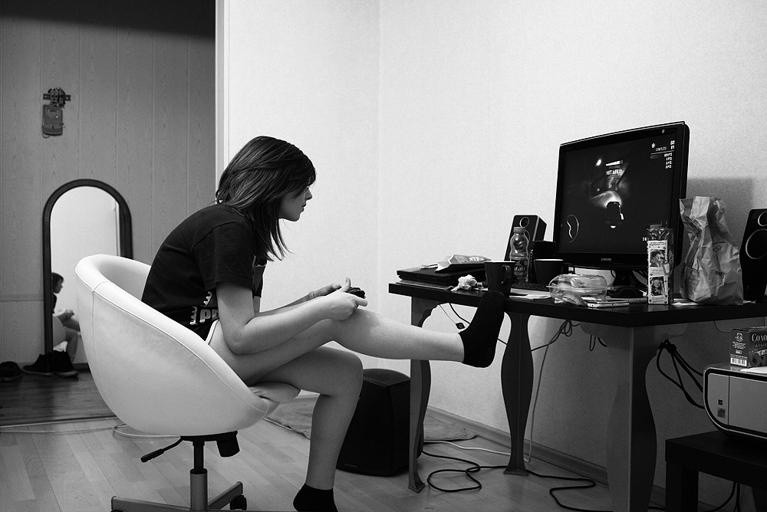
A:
[587,301,630,308]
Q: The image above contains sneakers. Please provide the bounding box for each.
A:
[0,360,23,383]
[22,350,79,379]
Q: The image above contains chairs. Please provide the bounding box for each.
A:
[70,253,298,511]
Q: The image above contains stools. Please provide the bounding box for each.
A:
[339,367,415,474]
[664,427,767,509]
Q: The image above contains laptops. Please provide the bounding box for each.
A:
[397,264,485,286]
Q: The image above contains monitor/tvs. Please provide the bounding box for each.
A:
[553,121,689,298]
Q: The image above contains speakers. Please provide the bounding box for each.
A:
[505,215,547,283]
[337,369,424,476]
[739,209,767,300]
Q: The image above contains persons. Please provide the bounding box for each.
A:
[141,136,511,512]
[51,273,81,362]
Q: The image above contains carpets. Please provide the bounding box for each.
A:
[0,368,117,426]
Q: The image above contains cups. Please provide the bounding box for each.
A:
[484,261,515,298]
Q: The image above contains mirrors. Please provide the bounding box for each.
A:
[42,178,136,367]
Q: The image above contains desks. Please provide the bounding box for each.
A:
[388,265,767,509]
